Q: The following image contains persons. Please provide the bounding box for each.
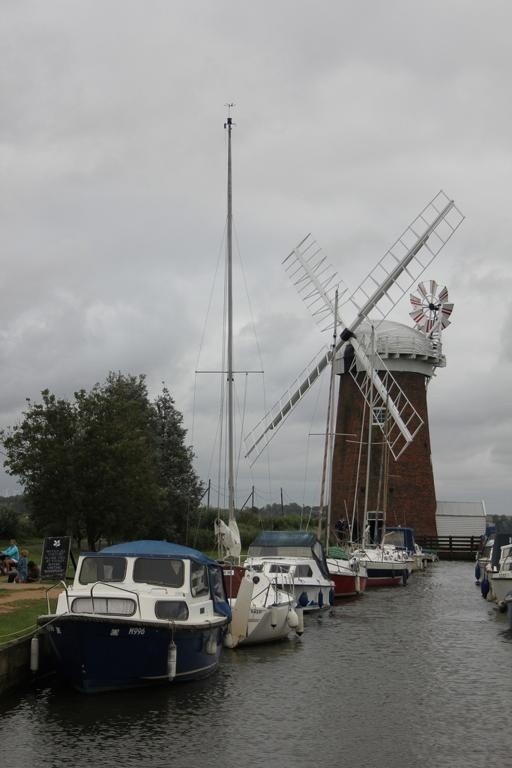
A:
[0,540,40,583]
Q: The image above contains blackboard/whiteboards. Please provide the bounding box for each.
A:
[40,535,72,580]
